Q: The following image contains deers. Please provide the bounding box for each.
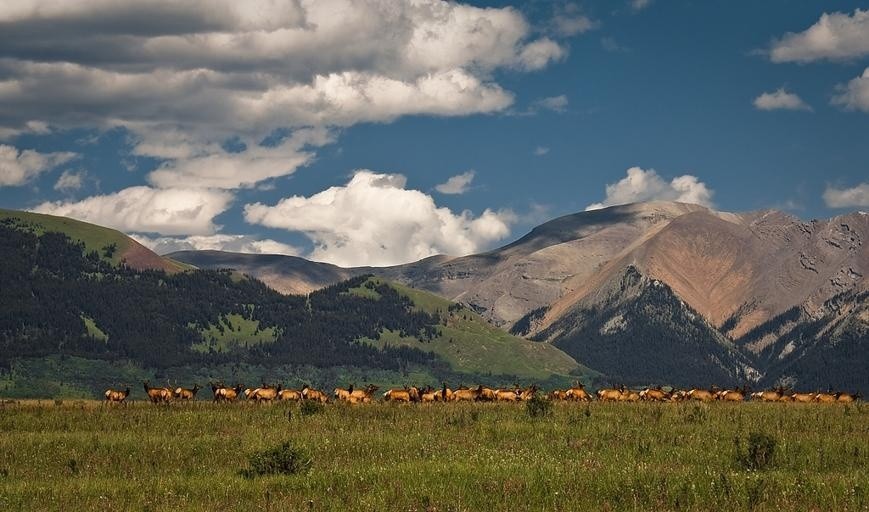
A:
[103,378,862,404]
